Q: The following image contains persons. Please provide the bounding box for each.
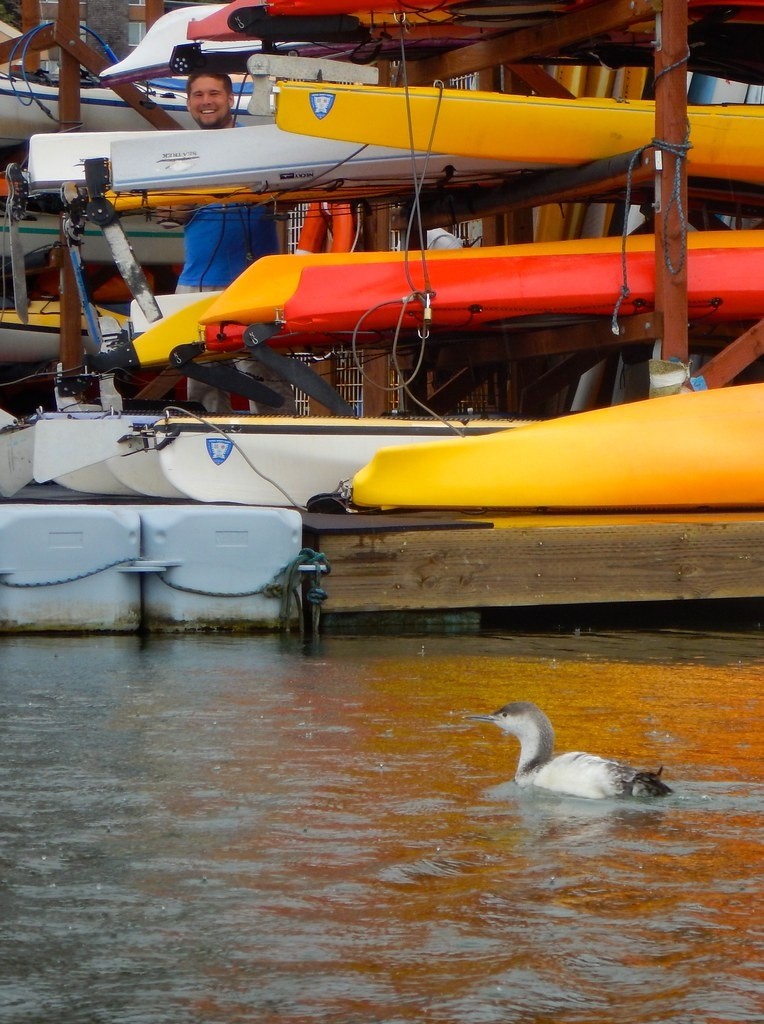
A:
[173,73,297,414]
[395,226,464,249]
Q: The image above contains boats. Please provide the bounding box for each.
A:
[0,0,764,517]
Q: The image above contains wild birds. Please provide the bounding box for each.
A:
[464,701,676,800]
